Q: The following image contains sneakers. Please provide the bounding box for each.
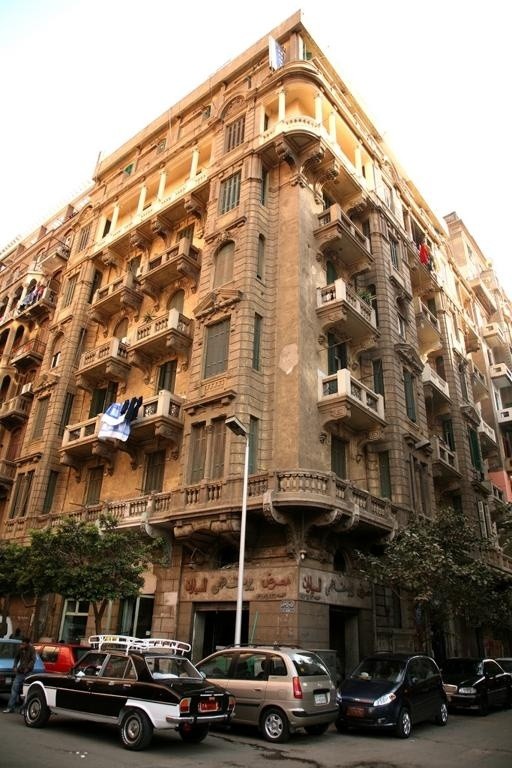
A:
[3,708,14,713]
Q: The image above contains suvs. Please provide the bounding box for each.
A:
[194,644,339,743]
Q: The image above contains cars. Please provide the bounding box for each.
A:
[494,658,511,675]
[29,636,114,677]
[438,657,511,716]
[0,638,46,703]
[334,650,448,738]
[20,634,237,751]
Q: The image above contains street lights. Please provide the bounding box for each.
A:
[224,415,249,648]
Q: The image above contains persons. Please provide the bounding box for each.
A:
[4,637,37,715]
